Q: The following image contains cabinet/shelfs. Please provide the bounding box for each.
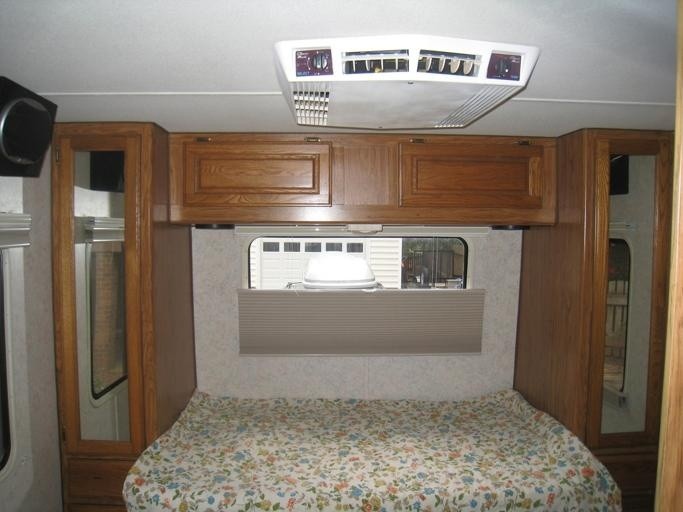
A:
[50,122,197,511]
[513,126,671,512]
[168,131,559,227]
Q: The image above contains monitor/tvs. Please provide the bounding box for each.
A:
[412,249,455,279]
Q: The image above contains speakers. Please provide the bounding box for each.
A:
[610,154,630,196]
[90,151,124,192]
[0,76,58,178]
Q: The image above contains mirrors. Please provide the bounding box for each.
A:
[586,131,671,451]
[62,131,144,457]
[247,236,469,290]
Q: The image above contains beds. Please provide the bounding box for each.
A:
[123,388,622,512]
